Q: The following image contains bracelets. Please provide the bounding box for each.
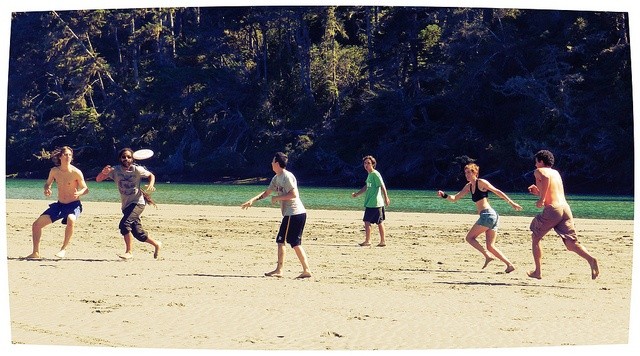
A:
[441,193,448,199]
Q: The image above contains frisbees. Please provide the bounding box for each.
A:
[133,149,154,160]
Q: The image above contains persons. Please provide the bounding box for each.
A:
[527,150,599,279]
[26,147,88,258]
[438,164,523,272]
[352,156,390,246]
[96,148,161,260]
[241,152,314,277]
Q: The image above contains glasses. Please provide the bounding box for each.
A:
[62,153,73,159]
[364,162,372,164]
[121,156,132,159]
